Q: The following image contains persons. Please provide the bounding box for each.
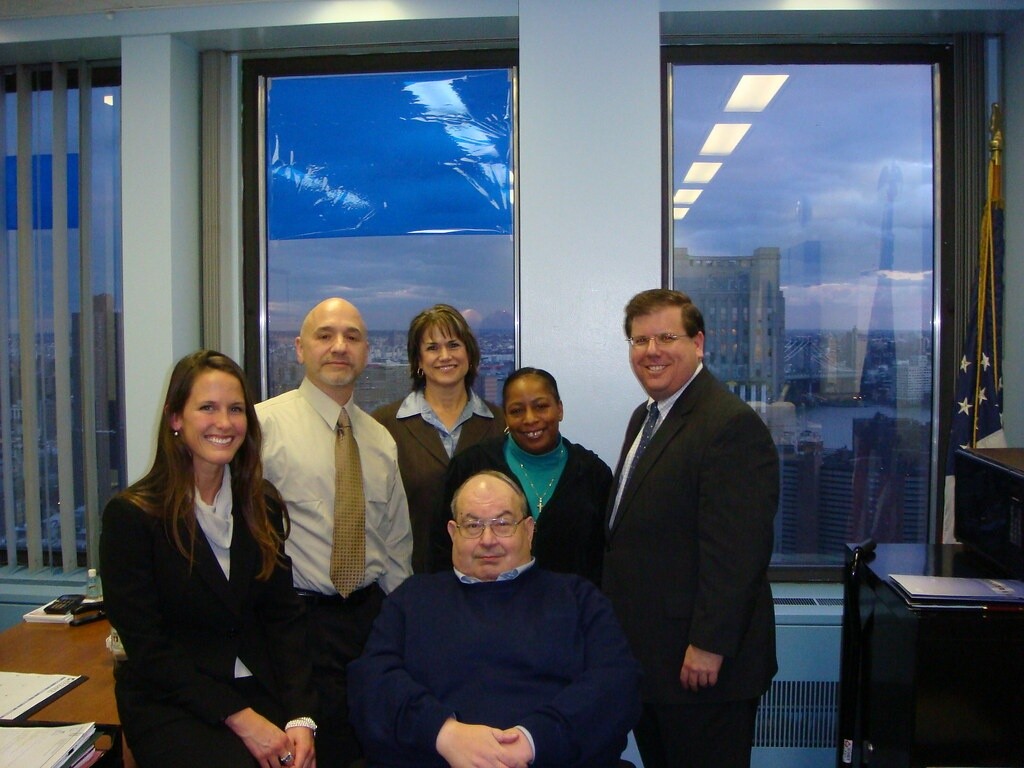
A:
[251,299,414,768]
[427,367,613,591]
[601,288,781,768]
[99,350,366,767]
[367,302,506,576]
[345,469,645,767]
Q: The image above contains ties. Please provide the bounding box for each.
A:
[329,407,366,599]
[622,400,659,491]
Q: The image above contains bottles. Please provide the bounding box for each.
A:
[85,568,102,599]
[111,622,128,661]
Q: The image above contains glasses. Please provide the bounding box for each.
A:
[456,516,524,538]
[626,333,689,349]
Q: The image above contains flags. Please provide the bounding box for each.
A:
[940,158,1009,544]
[846,200,910,543]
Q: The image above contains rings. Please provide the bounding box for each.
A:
[281,752,292,764]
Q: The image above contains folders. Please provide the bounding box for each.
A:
[890,573,1024,603]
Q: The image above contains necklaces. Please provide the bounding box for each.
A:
[510,447,564,513]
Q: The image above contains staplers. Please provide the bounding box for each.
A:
[69,601,105,627]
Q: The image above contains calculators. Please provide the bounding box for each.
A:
[43,594,86,614]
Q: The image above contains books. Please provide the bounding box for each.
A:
[887,573,1024,614]
